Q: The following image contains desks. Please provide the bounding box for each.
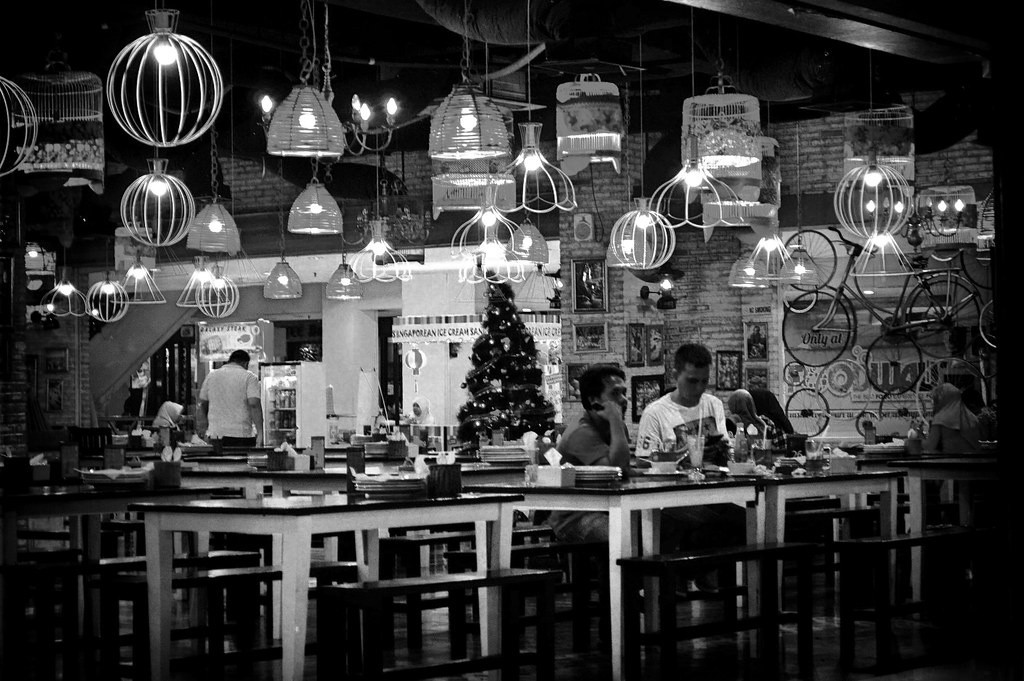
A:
[22,444,994,681]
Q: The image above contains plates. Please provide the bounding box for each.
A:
[480,446,530,463]
[863,444,904,453]
[351,477,425,500]
[573,465,623,482]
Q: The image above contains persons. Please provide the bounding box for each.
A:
[726,385,794,439]
[374,399,401,428]
[749,326,762,338]
[123,370,148,416]
[151,400,185,433]
[636,344,734,592]
[922,385,982,457]
[544,364,634,551]
[199,349,264,447]
[412,398,434,427]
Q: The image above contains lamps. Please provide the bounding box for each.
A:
[0,0,1024,322]
[405,343,426,394]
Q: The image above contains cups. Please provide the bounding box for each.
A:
[105,445,124,471]
[153,461,182,488]
[387,441,405,459]
[753,438,772,470]
[171,432,185,449]
[688,435,706,473]
[345,445,365,494]
[805,440,832,477]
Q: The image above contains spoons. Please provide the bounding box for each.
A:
[161,445,182,461]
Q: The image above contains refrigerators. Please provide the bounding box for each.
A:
[258,361,326,447]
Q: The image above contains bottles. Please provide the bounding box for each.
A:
[734,423,748,463]
[271,388,295,429]
[158,426,177,445]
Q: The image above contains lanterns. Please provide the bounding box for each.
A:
[406,348,426,395]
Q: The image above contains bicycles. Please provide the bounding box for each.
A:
[781,224,985,368]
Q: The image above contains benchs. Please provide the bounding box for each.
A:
[0,521,967,681]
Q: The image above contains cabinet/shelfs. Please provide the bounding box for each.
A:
[258,360,328,448]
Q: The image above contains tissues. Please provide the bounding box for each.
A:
[524,447,576,487]
[904,428,922,448]
[29,452,50,481]
[152,445,182,490]
[273,441,311,472]
[522,430,540,464]
[403,455,430,485]
[831,447,857,476]
[400,432,419,457]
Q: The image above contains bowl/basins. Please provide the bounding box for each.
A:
[651,462,678,473]
[727,461,753,474]
